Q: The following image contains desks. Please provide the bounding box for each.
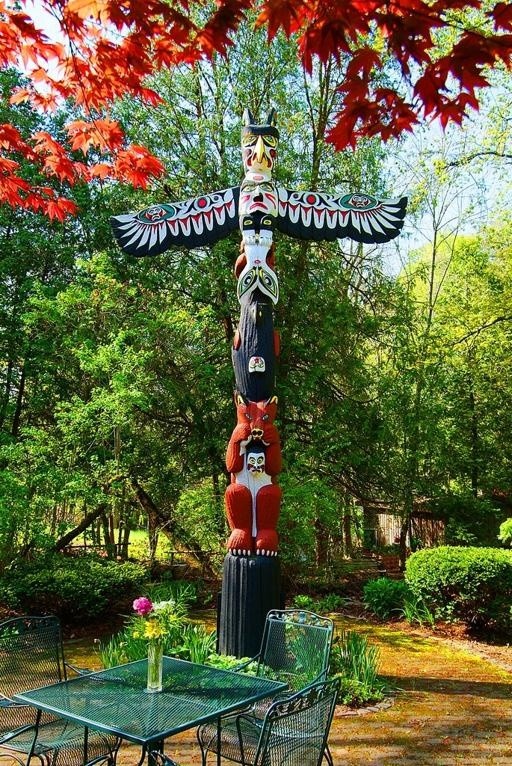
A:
[13,652,292,766]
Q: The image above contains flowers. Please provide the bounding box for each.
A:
[119,595,188,641]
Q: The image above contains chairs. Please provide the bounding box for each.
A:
[197,609,338,764]
[1,613,129,764]
[189,676,340,764]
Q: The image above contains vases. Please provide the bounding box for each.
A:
[143,644,165,694]
